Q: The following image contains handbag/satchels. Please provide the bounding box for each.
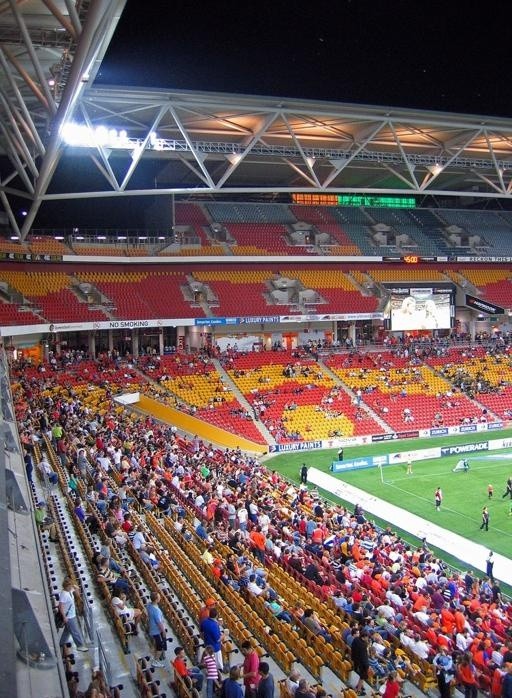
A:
[55,602,73,629]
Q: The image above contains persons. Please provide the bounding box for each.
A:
[392,297,440,330]
[7,325,511,698]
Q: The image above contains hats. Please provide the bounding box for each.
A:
[37,501,46,507]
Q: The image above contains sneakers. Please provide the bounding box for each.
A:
[76,645,88,652]
[152,660,166,668]
